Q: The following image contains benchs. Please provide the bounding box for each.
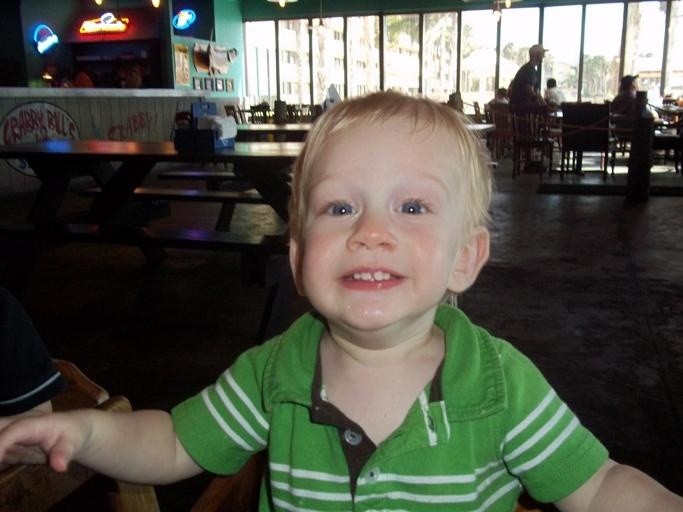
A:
[0,170,294,288]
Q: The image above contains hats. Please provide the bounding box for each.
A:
[622,74,639,86]
[529,44,548,55]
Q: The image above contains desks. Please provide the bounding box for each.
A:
[0,137,306,232]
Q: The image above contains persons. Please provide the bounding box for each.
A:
[30,56,149,88]
[488,47,682,173]
[0,280,67,468]
[0,87,682,512]
